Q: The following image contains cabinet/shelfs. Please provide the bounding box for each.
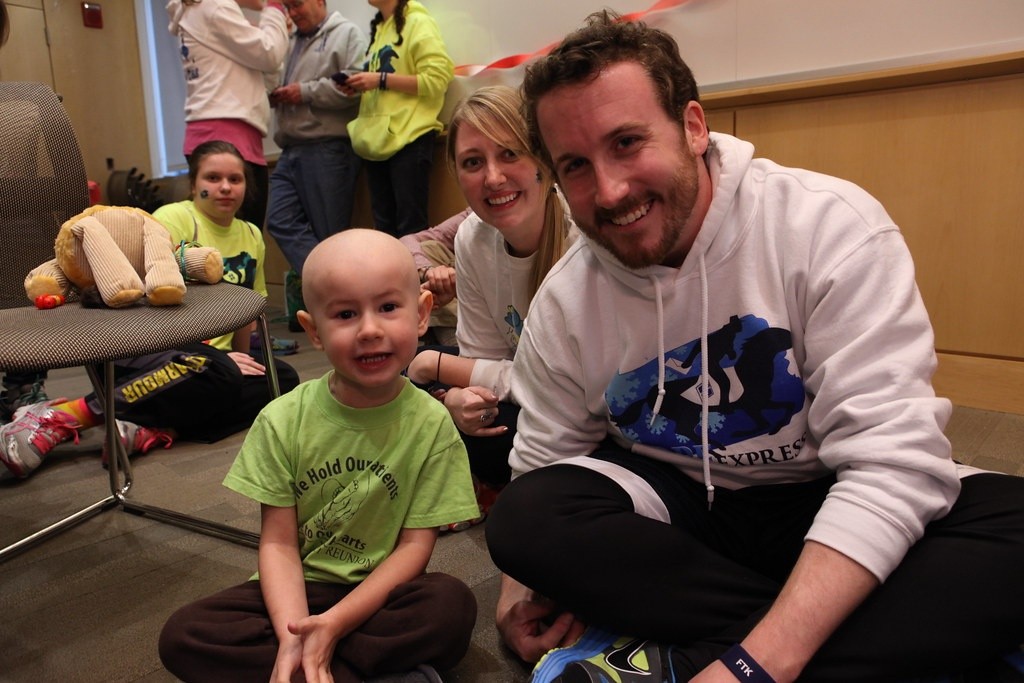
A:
[265,52,1022,416]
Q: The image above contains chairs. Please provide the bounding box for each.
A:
[1,81,283,562]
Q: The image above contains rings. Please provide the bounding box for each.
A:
[480,409,493,423]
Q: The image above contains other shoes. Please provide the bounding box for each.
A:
[284,269,308,332]
[366,664,442,683]
[438,475,497,531]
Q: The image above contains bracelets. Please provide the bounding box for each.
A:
[436,351,442,383]
[718,642,777,683]
[379,71,387,90]
[422,266,436,283]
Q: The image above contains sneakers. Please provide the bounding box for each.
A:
[526,625,676,683]
[0,397,83,479]
[102,414,176,470]
[249,329,300,356]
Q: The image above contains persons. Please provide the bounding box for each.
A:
[398,84,581,534]
[165,0,454,356]
[158,228,480,683]
[482,8,1024,683]
[0,140,301,477]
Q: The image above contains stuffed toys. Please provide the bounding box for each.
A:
[24,204,223,309]
